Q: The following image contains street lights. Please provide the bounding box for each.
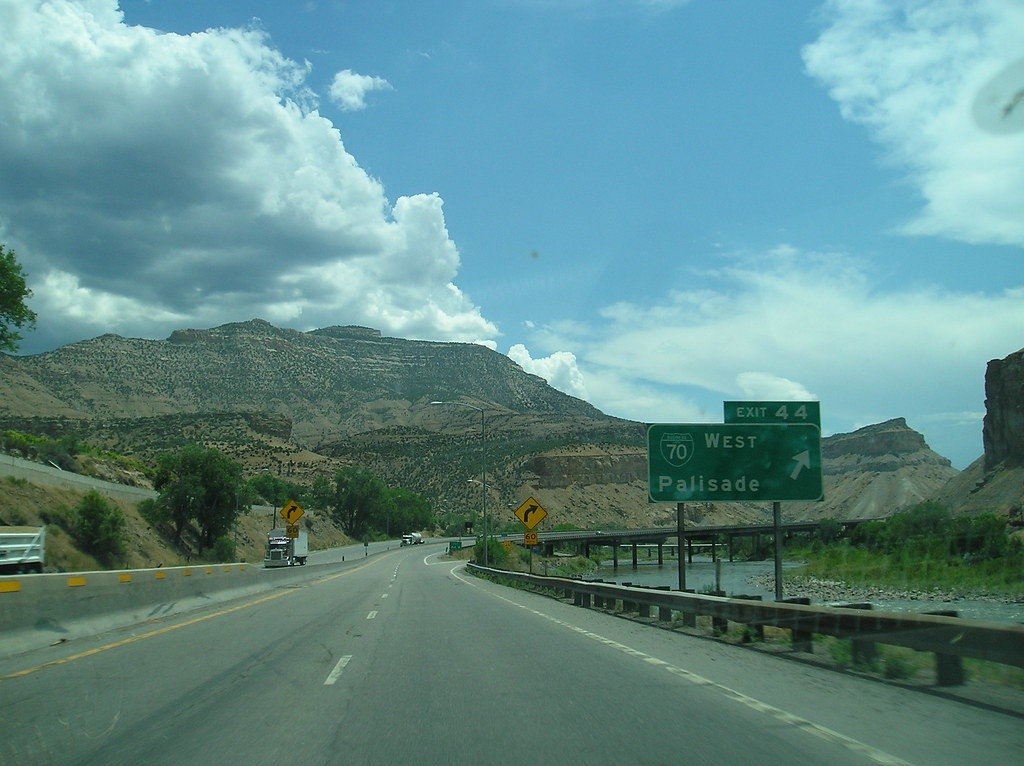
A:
[430,401,488,568]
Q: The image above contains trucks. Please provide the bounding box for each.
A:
[264,528,309,567]
[0,525,45,575]
[400,532,425,547]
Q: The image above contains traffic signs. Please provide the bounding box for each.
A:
[647,423,823,502]
